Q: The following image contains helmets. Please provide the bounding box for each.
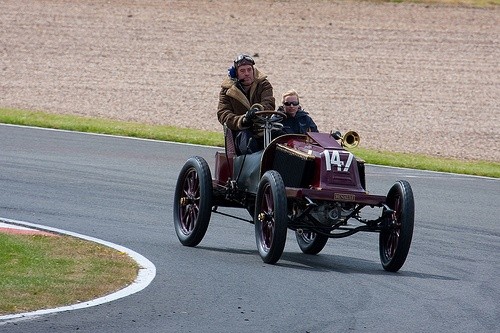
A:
[234,54,255,69]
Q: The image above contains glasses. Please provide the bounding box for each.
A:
[283,101,299,106]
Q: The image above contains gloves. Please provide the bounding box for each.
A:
[242,109,260,127]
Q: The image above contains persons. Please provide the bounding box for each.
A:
[272,90,319,141]
[217,54,276,155]
